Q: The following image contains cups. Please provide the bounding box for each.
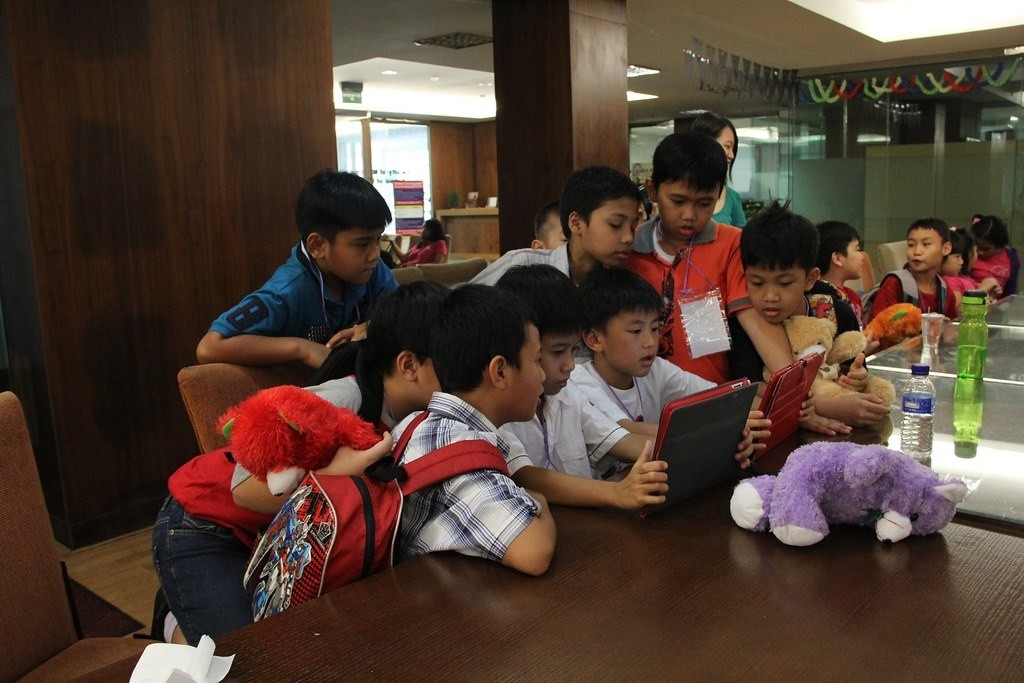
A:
[921,347,939,369]
[921,313,945,348]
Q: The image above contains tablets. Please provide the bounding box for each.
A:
[748,351,818,460]
[640,379,751,519]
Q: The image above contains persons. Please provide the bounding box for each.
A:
[197,167,400,368]
[531,197,568,250]
[816,214,1012,355]
[444,165,646,290]
[378,219,449,270]
[689,112,748,229]
[150,256,816,646]
[607,136,853,435]
[727,197,890,428]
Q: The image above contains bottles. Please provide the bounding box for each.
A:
[954,287,988,378]
[951,376,983,458]
[900,363,937,462]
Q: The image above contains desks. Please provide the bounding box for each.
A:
[192,296,1024,683]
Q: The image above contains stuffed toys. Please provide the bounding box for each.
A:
[731,441,970,546]
[761,316,898,409]
[213,384,382,497]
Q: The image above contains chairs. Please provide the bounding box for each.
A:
[0,389,169,683]
[377,232,489,288]
[178,361,317,455]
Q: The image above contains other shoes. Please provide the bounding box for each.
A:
[151,586,171,642]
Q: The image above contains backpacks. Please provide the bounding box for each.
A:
[242,411,512,622]
[804,278,850,338]
[860,268,947,326]
[306,339,384,430]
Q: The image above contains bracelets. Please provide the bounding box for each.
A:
[389,240,394,245]
